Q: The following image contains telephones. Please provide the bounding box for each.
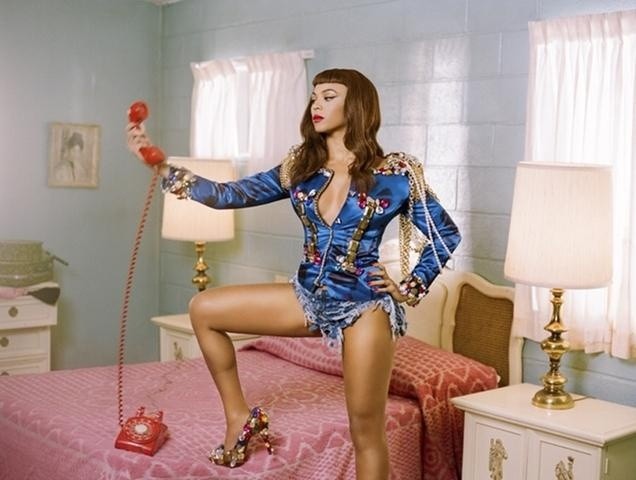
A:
[128,100,166,166]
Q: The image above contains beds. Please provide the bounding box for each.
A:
[0,237,526,480]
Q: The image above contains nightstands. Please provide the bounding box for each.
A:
[148,312,260,362]
[446,381,636,480]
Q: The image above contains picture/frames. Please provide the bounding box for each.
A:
[48,124,99,189]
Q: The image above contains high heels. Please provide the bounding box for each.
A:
[208,407,275,468]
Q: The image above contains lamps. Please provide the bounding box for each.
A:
[159,156,235,292]
[500,161,614,410]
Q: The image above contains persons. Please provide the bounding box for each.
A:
[127,69,462,479]
[57,130,88,182]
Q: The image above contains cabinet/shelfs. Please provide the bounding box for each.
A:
[0,282,59,376]
